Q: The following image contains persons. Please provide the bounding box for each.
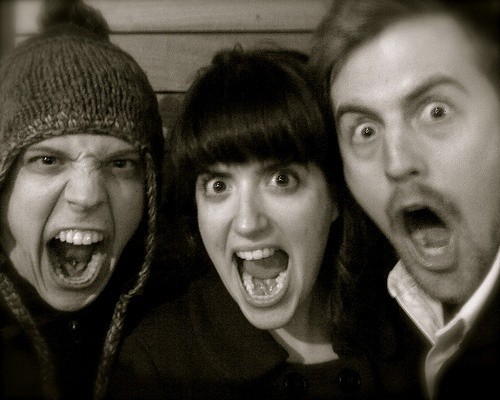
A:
[99,43,427,400]
[310,1,499,400]
[1,0,168,400]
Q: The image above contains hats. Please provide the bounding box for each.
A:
[0,0,166,399]
